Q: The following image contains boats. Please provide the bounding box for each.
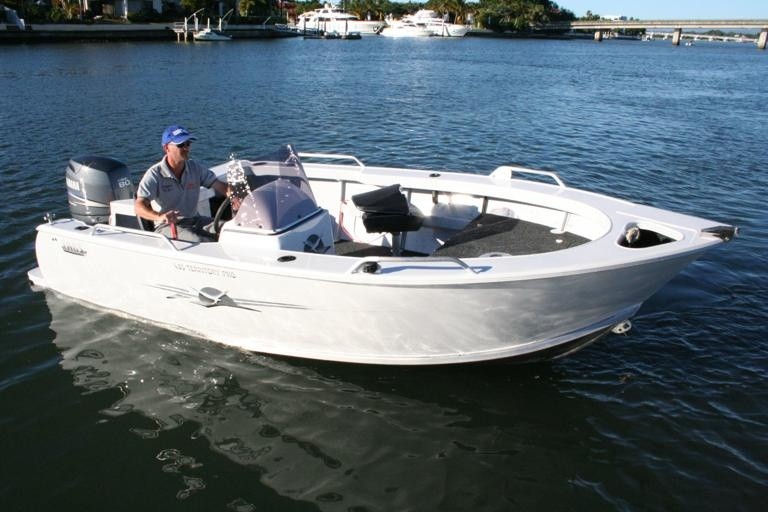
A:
[25,130,744,376]
[295,2,386,36]
[192,27,234,42]
[379,15,436,38]
[404,8,470,37]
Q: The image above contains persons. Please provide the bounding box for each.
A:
[440,8,481,25]
[135,124,243,242]
[356,8,389,21]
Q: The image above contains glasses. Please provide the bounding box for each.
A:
[176,141,192,147]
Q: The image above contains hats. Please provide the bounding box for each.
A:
[161,125,198,145]
